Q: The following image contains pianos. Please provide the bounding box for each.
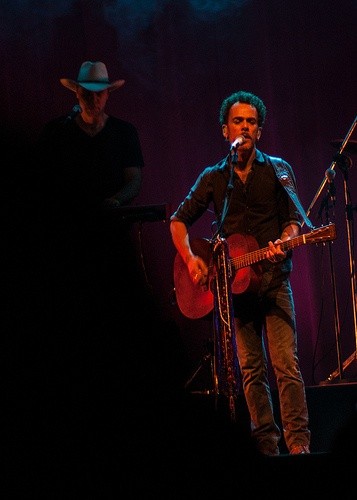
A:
[96,202,170,226]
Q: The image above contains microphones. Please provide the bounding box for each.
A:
[230,134,246,149]
[65,104,80,122]
[325,169,336,206]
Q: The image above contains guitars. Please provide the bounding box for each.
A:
[173,222,336,319]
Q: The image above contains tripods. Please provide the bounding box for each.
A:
[185,157,357,394]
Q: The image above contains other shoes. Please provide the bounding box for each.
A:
[288,440,310,454]
[256,440,280,456]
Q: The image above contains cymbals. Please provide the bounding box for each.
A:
[331,138,357,156]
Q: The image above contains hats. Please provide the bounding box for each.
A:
[60,61,125,93]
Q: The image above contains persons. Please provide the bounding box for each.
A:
[169,91,310,455]
[37,61,145,207]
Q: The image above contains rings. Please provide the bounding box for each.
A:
[195,274,197,279]
